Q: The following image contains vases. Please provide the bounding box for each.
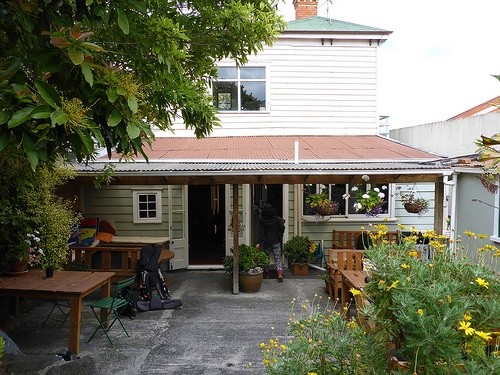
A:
[10,260,25,272]
[290,261,309,276]
[404,203,423,214]
[316,201,341,214]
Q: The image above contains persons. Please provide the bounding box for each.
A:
[256,203,286,283]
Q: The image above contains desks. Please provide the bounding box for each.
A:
[0,266,117,357]
[340,267,377,322]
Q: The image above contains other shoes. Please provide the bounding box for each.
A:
[278,274,283,282]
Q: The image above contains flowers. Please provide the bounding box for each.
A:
[355,188,388,216]
[281,234,319,267]
[392,184,430,216]
[305,191,331,207]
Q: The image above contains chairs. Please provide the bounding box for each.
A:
[42,298,72,330]
[84,273,136,349]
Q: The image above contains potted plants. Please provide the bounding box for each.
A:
[31,187,84,277]
[224,242,269,292]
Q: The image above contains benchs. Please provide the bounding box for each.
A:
[324,249,374,307]
[333,229,402,251]
